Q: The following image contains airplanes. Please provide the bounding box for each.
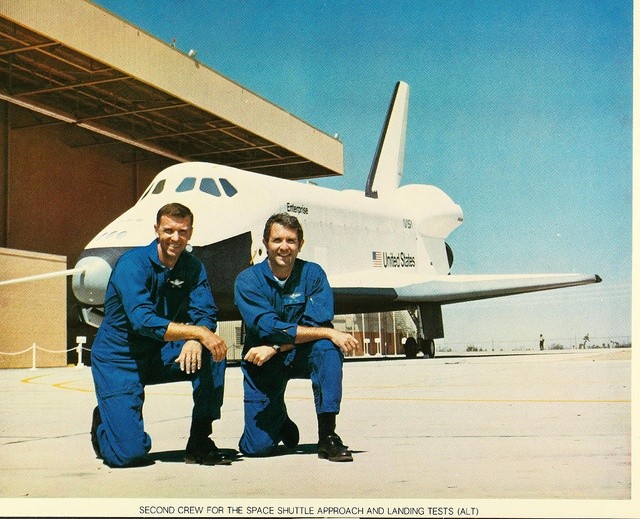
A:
[71,79,603,358]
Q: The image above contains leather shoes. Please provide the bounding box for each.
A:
[317,434,355,462]
[90,405,105,459]
[281,403,299,448]
[184,439,232,466]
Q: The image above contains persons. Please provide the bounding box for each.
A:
[91,202,231,467]
[233,213,361,462]
[540,334,544,350]
[584,334,590,349]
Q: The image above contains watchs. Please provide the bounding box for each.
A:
[269,344,280,353]
